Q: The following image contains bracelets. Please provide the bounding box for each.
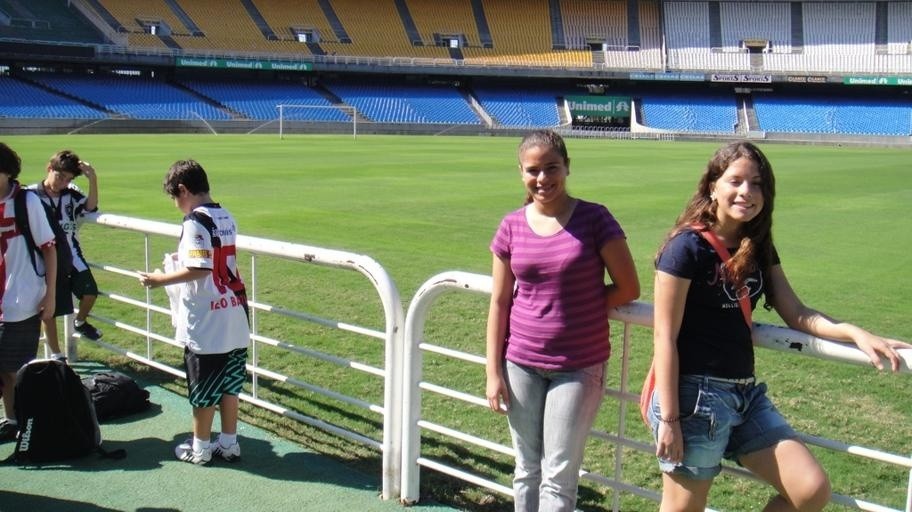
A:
[659,415,680,422]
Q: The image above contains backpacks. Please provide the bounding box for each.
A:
[13,359,102,464]
[14,189,73,279]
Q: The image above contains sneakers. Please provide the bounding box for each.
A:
[175,442,213,465]
[209,438,241,462]
[72,318,102,340]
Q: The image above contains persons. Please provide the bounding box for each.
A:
[140,158,251,467]
[642,141,912,511]
[21,150,104,365]
[485,126,641,510]
[1,144,59,444]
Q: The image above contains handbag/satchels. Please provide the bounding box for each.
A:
[80,373,150,420]
[638,350,656,427]
